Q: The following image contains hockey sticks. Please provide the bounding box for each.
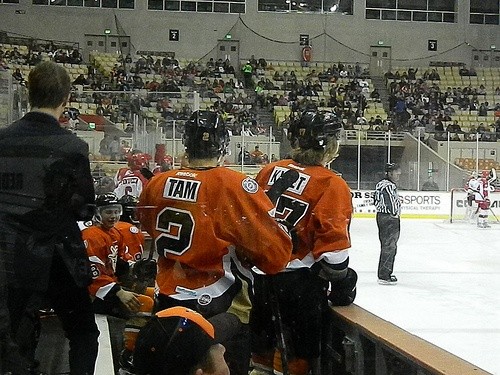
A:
[265,170,299,375]
[477,191,500,224]
[141,168,156,259]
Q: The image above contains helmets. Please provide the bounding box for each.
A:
[94,193,122,222]
[287,110,341,151]
[385,163,400,172]
[181,109,230,160]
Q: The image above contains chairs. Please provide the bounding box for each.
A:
[0,44,123,131]
[254,62,387,129]
[392,67,500,141]
[90,50,252,118]
[455,158,500,174]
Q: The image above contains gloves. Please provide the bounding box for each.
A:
[327,266,358,305]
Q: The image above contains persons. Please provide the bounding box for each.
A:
[80,191,154,375]
[135,111,293,375]
[374,163,401,285]
[242,56,500,142]
[0,43,281,228]
[0,63,100,375]
[247,112,357,375]
[422,176,439,191]
[132,307,238,375]
[464,168,497,228]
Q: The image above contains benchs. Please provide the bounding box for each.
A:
[75,220,113,375]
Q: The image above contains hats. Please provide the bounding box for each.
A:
[133,307,214,375]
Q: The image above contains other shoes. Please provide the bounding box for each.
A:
[378,275,397,285]
[477,222,492,229]
